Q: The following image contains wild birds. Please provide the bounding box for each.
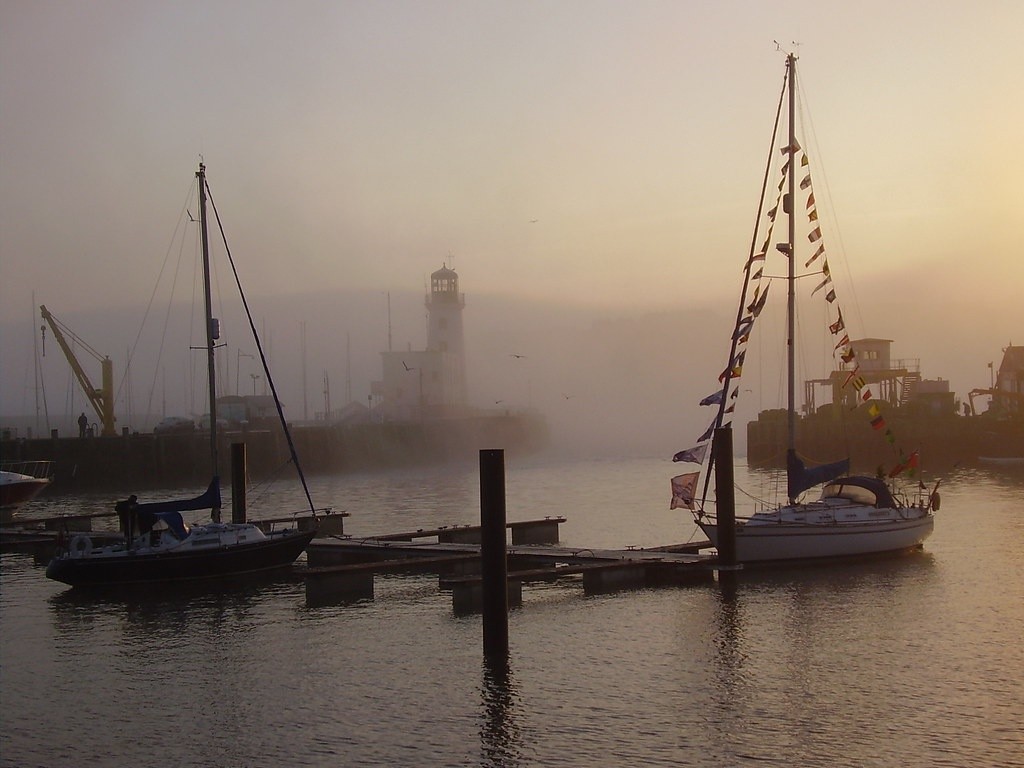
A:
[742,388,751,393]
[528,219,539,223]
[561,392,573,399]
[185,208,202,224]
[401,360,416,372]
[495,400,502,404]
[509,354,525,359]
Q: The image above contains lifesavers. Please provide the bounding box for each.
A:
[69,535,93,555]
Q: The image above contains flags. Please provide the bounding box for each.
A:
[889,447,920,476]
[696,415,718,442]
[866,400,879,416]
[884,430,897,445]
[700,389,725,406]
[870,415,886,432]
[673,443,710,464]
[920,481,926,490]
[670,472,700,511]
[723,402,737,414]
[723,420,733,429]
[717,138,874,401]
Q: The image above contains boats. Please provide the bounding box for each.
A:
[0,456,58,508]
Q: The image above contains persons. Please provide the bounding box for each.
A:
[77,412,88,439]
[963,402,970,416]
[115,495,142,541]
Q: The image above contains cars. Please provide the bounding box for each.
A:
[198,412,231,434]
[153,416,196,436]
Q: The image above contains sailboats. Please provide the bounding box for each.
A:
[44,151,348,589]
[668,39,945,566]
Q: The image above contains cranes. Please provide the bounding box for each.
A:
[40,302,119,438]
